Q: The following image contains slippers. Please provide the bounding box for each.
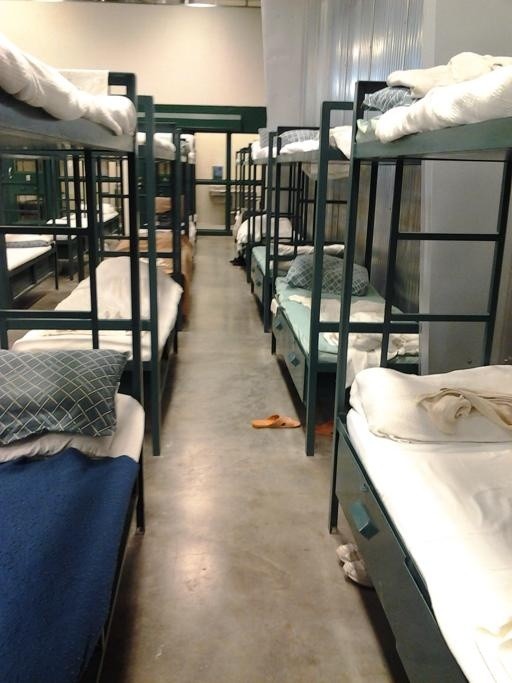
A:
[230,256,247,266]
[315,419,334,437]
[251,415,302,429]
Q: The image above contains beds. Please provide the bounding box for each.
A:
[227,42,512,681]
[2,37,198,681]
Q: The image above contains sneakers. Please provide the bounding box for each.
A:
[342,560,373,587]
[337,539,360,562]
